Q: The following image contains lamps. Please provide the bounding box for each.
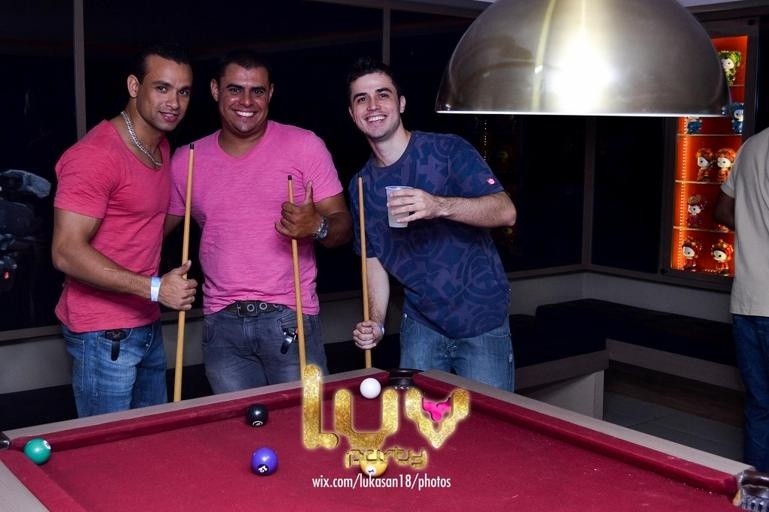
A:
[434,0,732,118]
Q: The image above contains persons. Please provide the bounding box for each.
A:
[50,50,199,417]
[166,56,352,396]
[346,58,517,393]
[712,127,768,478]
[680,49,746,277]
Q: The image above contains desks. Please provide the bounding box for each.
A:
[0,368,756,512]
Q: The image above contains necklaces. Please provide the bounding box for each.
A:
[121,111,163,168]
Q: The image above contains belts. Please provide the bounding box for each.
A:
[223,298,287,318]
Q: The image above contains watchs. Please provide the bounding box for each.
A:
[377,322,386,334]
[315,215,329,240]
[149,277,162,302]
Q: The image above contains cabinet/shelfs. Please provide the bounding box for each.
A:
[670,35,748,278]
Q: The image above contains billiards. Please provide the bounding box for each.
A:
[25,439,51,464]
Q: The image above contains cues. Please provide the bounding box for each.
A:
[174,145,195,401]
[357,174,372,368]
[288,174,305,382]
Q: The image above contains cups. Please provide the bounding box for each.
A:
[384,185,413,228]
[687,115,703,134]
[718,50,742,86]
[730,103,743,134]
